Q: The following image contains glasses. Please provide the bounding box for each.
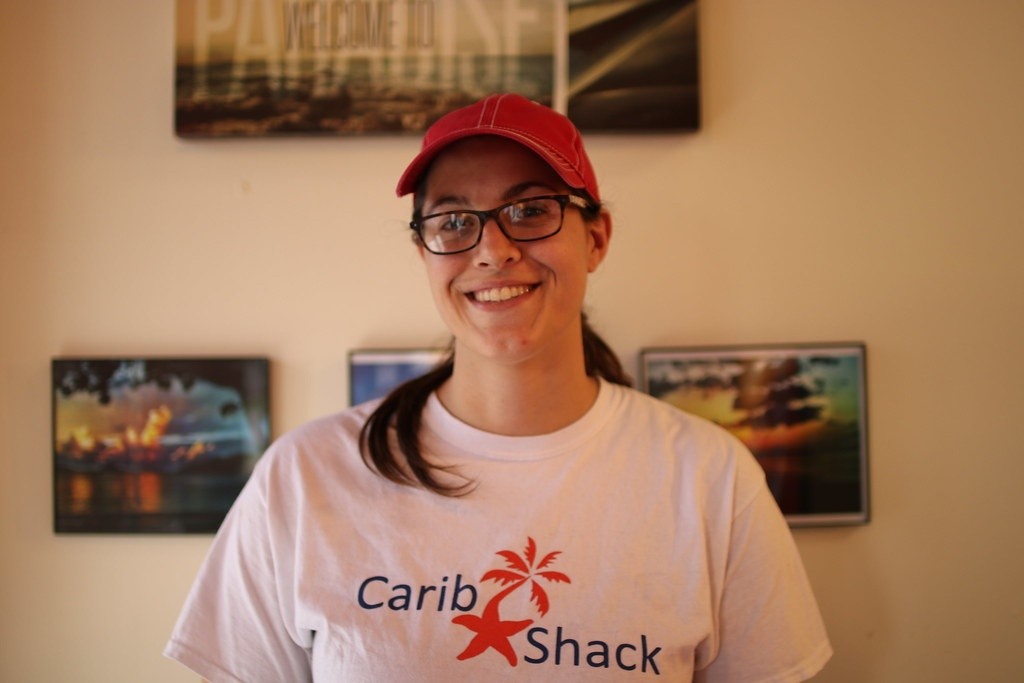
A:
[409,194,589,255]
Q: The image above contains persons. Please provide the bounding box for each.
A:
[162,90,836,683]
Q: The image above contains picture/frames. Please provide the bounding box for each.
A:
[640,339,873,529]
[50,356,275,536]
[343,346,455,406]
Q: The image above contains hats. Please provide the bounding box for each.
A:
[396,92,601,204]
